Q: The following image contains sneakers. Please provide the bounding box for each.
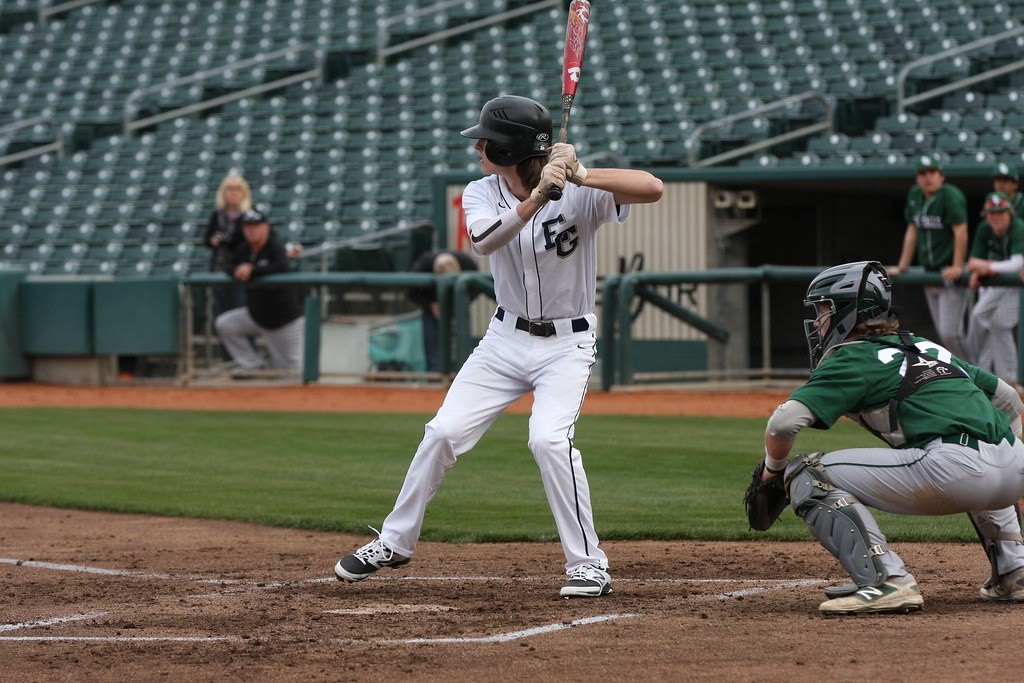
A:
[560,563,612,596]
[979,565,1024,603]
[819,573,924,615]
[334,525,411,583]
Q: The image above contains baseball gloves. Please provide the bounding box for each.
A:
[743,458,791,533]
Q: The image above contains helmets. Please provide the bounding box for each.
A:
[460,94,552,167]
[803,261,892,373]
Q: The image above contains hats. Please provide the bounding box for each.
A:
[983,192,1012,211]
[914,155,942,172]
[992,163,1019,181]
[243,210,265,224]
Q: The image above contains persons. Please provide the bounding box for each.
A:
[743,261,1024,615]
[203,172,306,381]
[885,154,1024,396]
[407,251,480,383]
[335,95,663,597]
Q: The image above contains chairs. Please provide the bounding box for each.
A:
[0,1,1024,305]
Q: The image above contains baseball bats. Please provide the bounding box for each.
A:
[545,0,591,201]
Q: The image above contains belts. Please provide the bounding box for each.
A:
[495,307,589,337]
[942,428,1015,451]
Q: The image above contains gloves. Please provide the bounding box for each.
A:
[546,142,587,187]
[530,161,566,206]
